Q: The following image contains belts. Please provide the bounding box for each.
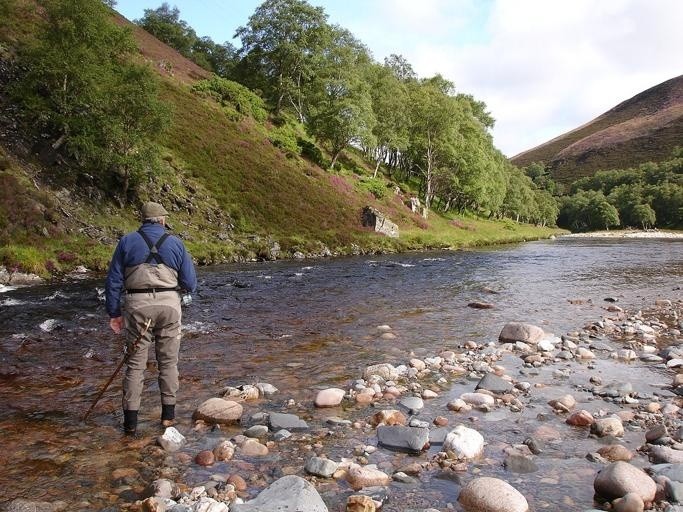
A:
[127,287,176,294]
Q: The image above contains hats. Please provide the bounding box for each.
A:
[142,202,170,217]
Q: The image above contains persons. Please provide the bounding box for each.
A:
[103,200,199,435]
